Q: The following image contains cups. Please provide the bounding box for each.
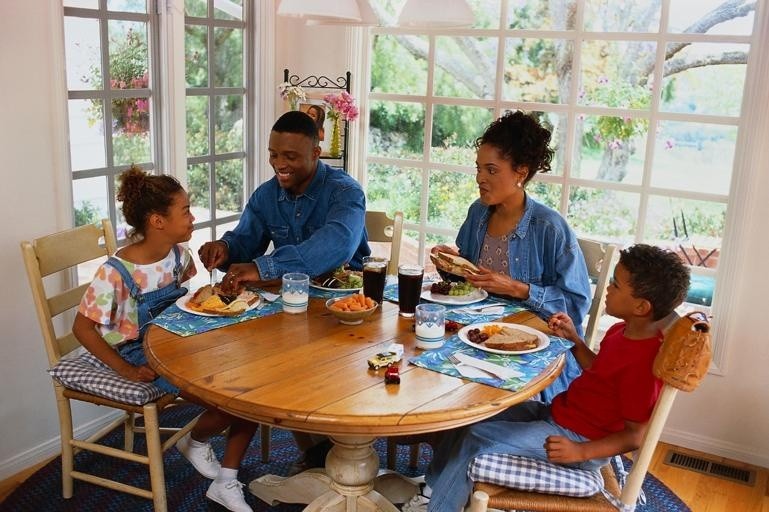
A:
[281,273,310,315]
[362,256,389,306]
[397,264,426,317]
[415,304,446,350]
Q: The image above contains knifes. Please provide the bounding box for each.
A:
[210,268,213,285]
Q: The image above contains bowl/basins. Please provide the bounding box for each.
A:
[325,297,379,326]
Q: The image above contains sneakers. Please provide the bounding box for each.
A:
[203,476,255,511]
[171,429,223,481]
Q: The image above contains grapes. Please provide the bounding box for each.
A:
[467,328,488,344]
[431,280,474,296]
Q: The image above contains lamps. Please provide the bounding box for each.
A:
[275,0,479,34]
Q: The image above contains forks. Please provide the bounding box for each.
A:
[467,303,508,312]
[443,350,505,383]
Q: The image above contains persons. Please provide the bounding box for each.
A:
[72,165,259,512]
[306,105,325,141]
[401,244,691,512]
[198,111,371,469]
[428,110,593,405]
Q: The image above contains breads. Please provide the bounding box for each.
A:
[485,327,540,351]
[430,252,481,277]
[191,282,258,315]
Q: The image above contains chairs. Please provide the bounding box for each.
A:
[407,226,616,484]
[257,209,406,466]
[461,312,710,511]
[17,217,231,512]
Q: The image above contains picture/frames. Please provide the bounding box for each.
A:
[282,93,339,160]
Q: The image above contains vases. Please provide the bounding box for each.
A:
[329,118,344,160]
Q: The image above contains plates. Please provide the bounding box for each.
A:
[176,290,261,317]
[418,280,488,305]
[309,280,363,292]
[457,322,551,355]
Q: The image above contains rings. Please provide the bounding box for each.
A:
[229,270,239,277]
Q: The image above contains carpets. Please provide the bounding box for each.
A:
[1,401,696,511]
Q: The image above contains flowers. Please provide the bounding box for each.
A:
[502,0,683,156]
[321,89,361,125]
[73,21,207,140]
[277,81,309,111]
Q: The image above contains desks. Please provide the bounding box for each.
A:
[676,235,723,268]
